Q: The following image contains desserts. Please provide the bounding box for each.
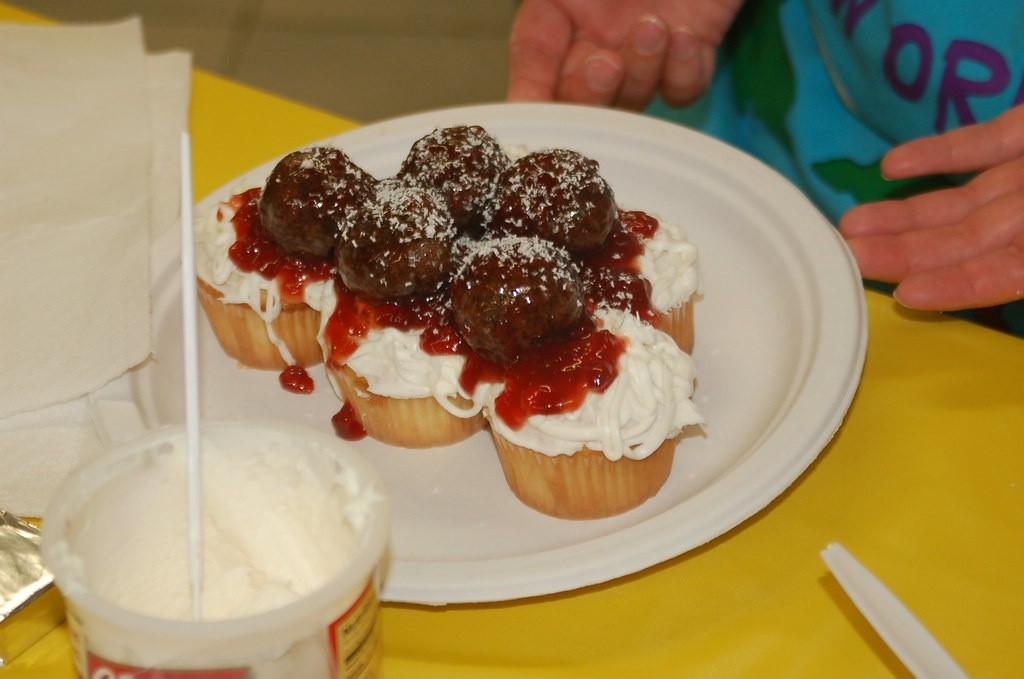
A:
[191,125,705,519]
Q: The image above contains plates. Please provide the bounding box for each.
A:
[137,101,867,604]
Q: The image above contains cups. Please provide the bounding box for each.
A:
[44,422,388,679]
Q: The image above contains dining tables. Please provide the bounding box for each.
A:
[0,4,1023,679]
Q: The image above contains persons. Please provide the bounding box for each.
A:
[505,0,1024,313]
